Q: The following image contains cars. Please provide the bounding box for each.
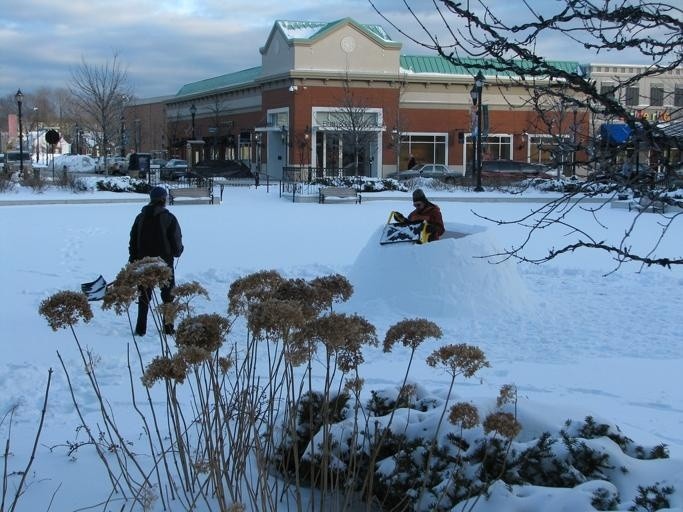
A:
[388,164,462,182]
[96,152,187,180]
[588,163,664,185]
[481,160,555,183]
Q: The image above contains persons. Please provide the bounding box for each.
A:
[394,189,445,244]
[128,186,184,337]
[408,154,417,169]
[128,146,140,180]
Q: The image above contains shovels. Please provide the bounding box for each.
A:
[81,275,118,301]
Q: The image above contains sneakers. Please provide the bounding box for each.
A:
[163,328,176,334]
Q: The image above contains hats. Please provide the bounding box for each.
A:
[150,187,167,201]
[413,189,426,202]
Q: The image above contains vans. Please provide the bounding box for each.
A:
[0,151,30,170]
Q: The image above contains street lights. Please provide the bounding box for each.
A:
[280,125,289,169]
[470,70,486,192]
[15,88,24,172]
[304,126,312,185]
[189,103,197,139]
[572,104,579,179]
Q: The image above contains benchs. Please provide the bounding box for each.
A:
[166,187,214,205]
[318,186,362,204]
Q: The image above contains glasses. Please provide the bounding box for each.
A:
[414,203,421,207]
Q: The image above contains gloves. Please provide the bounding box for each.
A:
[394,212,404,221]
